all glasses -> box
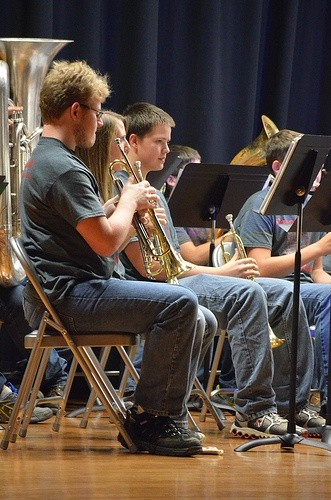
[79,104,103,119]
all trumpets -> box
[111,139,194,287]
[220,212,285,348]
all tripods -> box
[235,135,330,455]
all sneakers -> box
[117,414,202,455]
[231,409,308,441]
[279,409,325,436]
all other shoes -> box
[111,400,135,415]
[10,385,44,401]
[0,391,53,423]
[35,398,67,415]
[185,396,208,412]
[189,429,205,441]
[210,391,237,414]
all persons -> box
[230,129,331,426]
[0,107,70,424]
[160,144,326,438]
[18,60,220,456]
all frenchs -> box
[207,113,278,239]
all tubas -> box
[0,37,75,288]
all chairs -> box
[0,236,228,455]
